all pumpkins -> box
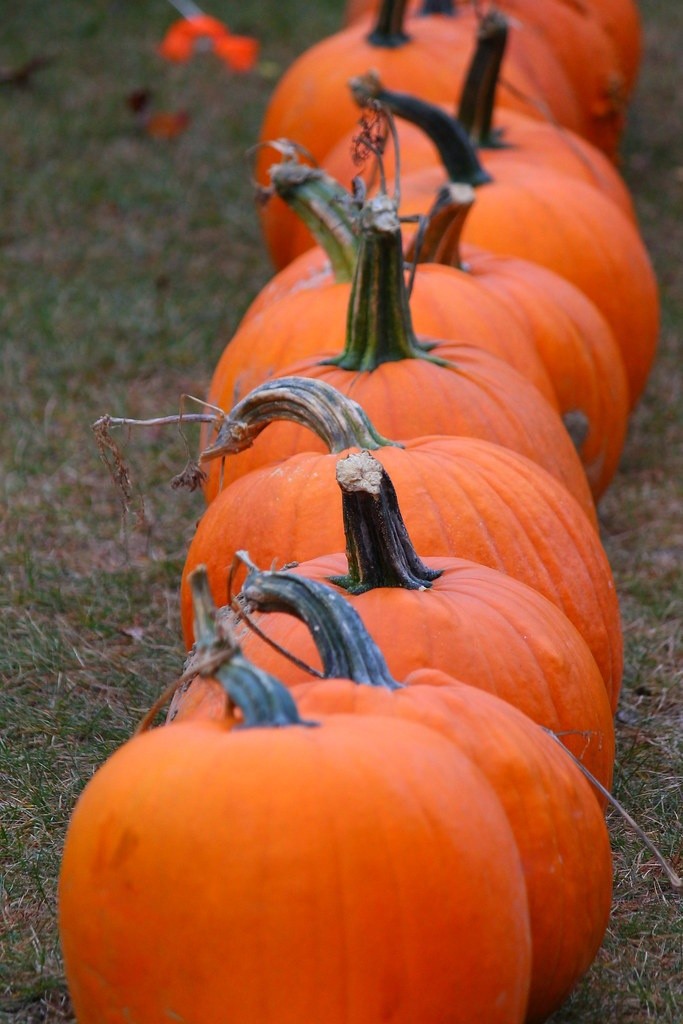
[59,0,660,1024]
